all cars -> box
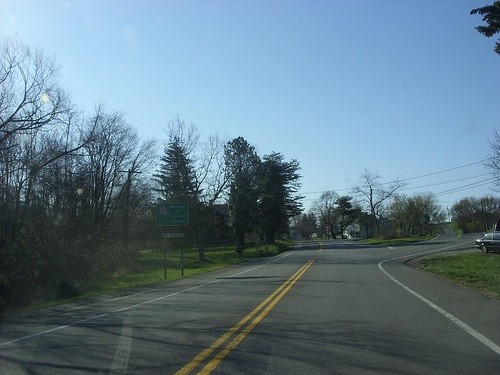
[476,231,500,255]
